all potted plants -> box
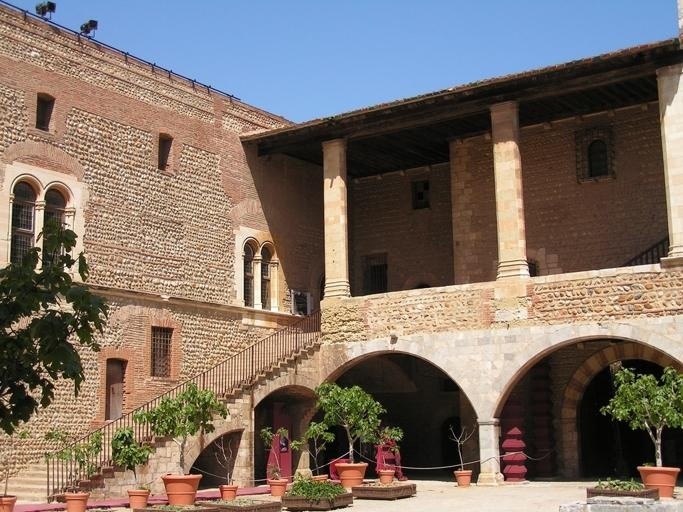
[584,476,661,501]
[260,426,288,497]
[214,435,238,501]
[110,426,154,510]
[281,383,417,509]
[448,425,473,488]
[43,428,104,511]
[596,363,683,498]
[132,384,230,506]
[1,416,34,511]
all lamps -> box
[77,19,98,40]
[34,0,56,23]
